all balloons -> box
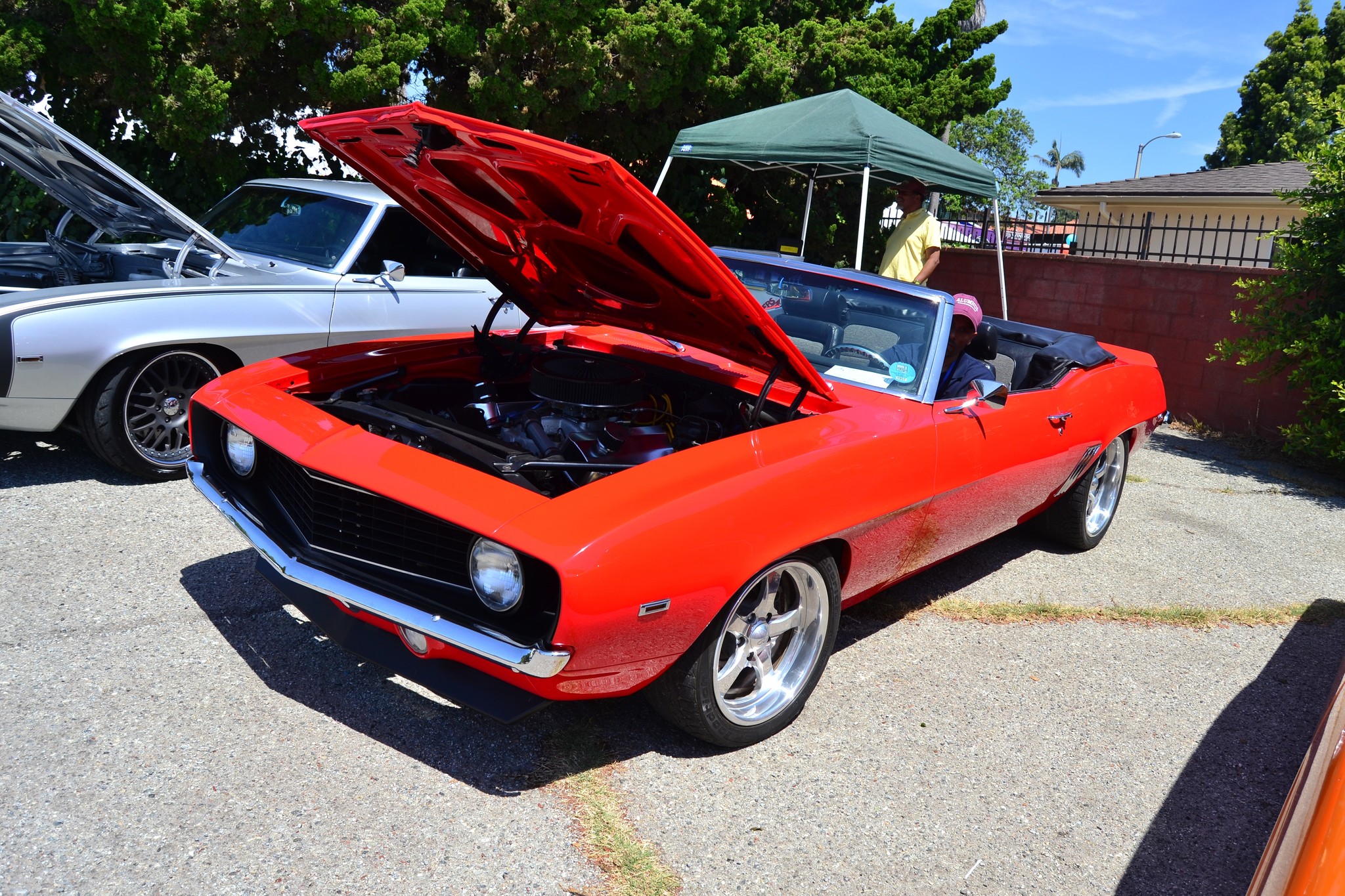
[1066,234,1076,245]
[1059,244,1070,254]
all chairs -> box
[772,284,851,361]
[921,312,1000,382]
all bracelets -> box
[912,279,921,285]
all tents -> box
[652,89,1009,321]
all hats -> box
[952,293,983,332]
[889,179,928,194]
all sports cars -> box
[0,88,583,485]
[181,99,1169,751]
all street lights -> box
[1134,131,1182,178]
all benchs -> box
[839,305,1057,387]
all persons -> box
[870,293,995,399]
[877,179,941,286]
